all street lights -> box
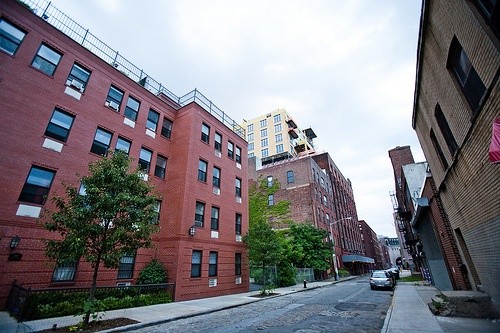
[329,217,352,281]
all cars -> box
[387,267,400,286]
[369,271,393,291]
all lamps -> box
[190,224,196,237]
[8,235,23,260]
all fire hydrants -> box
[303,280,307,288]
